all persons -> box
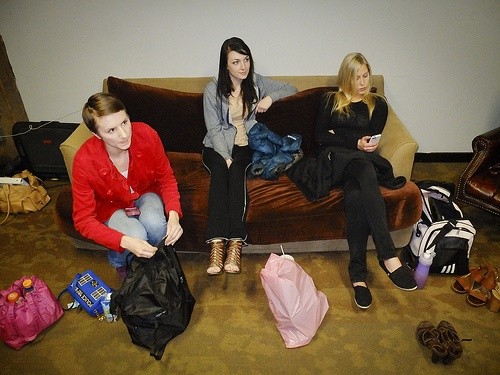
[316,52,417,309]
[203,37,300,274]
[72,92,184,273]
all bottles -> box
[22,279,34,296]
[414,252,434,289]
[7,290,24,305]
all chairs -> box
[455,125,500,215]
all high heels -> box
[438,321,473,358]
[416,321,453,364]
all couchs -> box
[58,75,423,255]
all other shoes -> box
[353,285,372,308]
[379,259,417,291]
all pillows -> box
[107,76,207,153]
[263,86,377,151]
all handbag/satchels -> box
[259,254,329,349]
[108,236,195,361]
[0,170,51,225]
[0,274,63,350]
[404,181,476,275]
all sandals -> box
[450,265,489,294]
[224,240,241,274]
[207,239,225,275]
[466,266,500,307]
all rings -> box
[147,256,148,258]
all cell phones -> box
[369,134,381,145]
